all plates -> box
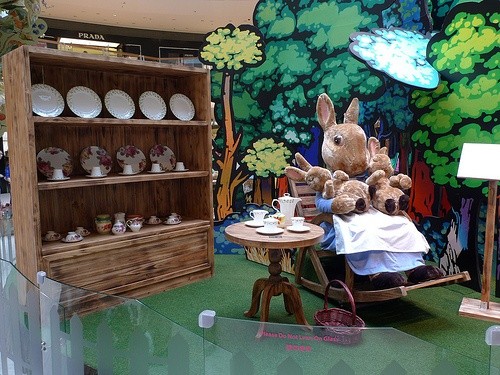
[163,221,181,224]
[169,94,195,120]
[37,147,73,177]
[104,90,135,119]
[80,146,113,175]
[116,145,146,173]
[86,175,107,178]
[147,171,165,173]
[42,236,62,241]
[139,91,167,120]
[145,220,161,224]
[118,172,137,175]
[79,232,91,237]
[31,84,65,117]
[245,220,264,227]
[173,169,189,171]
[47,177,70,180]
[287,226,310,232]
[150,144,176,170]
[257,227,284,234]
[66,86,102,118]
[62,237,83,242]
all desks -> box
[225,222,325,340]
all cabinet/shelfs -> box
[1,44,215,323]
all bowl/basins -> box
[149,216,159,223]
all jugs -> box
[126,218,144,232]
[272,193,302,224]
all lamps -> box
[456,143,500,323]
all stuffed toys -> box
[316,93,444,291]
[285,152,376,214]
[365,137,412,215]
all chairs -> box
[287,178,471,302]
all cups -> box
[66,232,81,240]
[171,213,182,219]
[151,164,162,171]
[91,167,103,176]
[76,227,88,234]
[52,169,64,178]
[123,165,132,173]
[263,218,278,230]
[167,216,179,223]
[45,231,60,239]
[291,217,305,228]
[176,162,184,170]
[250,210,269,221]
